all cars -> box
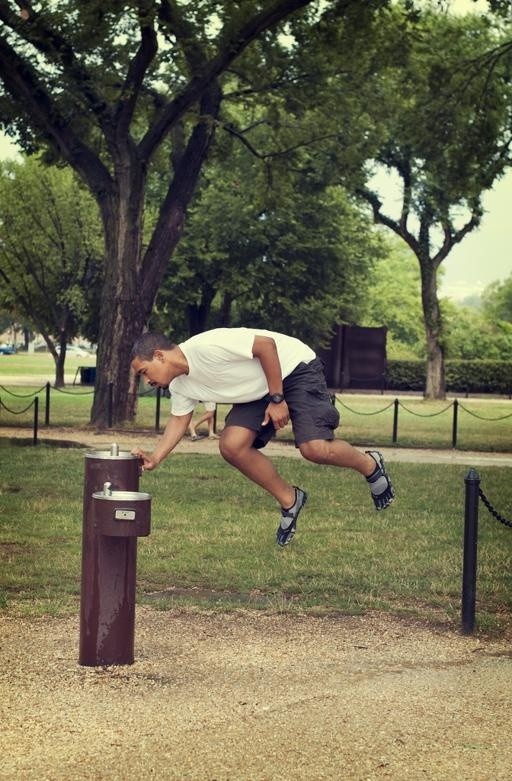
[55,344,89,359]
[0,344,16,353]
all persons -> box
[187,401,221,440]
[130,326,395,547]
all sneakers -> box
[363,450,396,512]
[188,427,221,442]
[274,486,307,549]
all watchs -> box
[270,393,285,404]
